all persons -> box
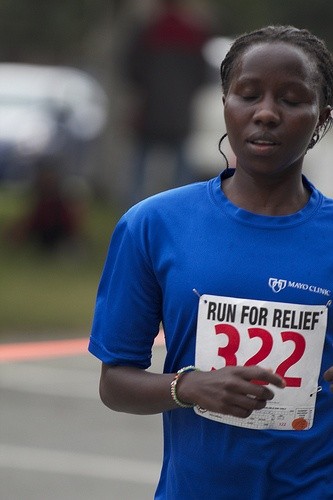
[99,25,333,500]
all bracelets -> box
[170,365,201,409]
